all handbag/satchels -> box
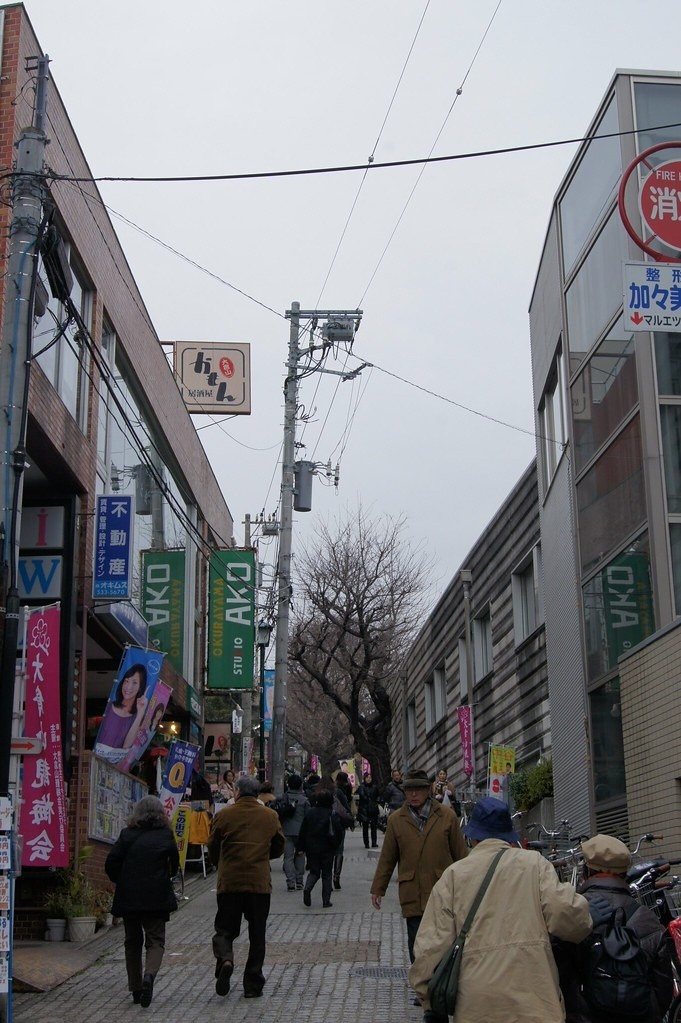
[442,792,461,818]
[333,791,348,821]
[427,933,471,1016]
[274,794,298,818]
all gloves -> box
[587,896,614,929]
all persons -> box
[410,797,593,1023]
[217,770,235,798]
[136,703,164,759]
[105,796,181,1008]
[341,761,350,774]
[98,664,150,750]
[433,769,456,810]
[355,774,381,848]
[259,771,355,907]
[209,777,286,997]
[550,833,674,1023]
[370,770,470,963]
[384,771,406,813]
[501,763,514,811]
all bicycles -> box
[510,809,681,1023]
[459,799,476,848]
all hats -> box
[582,833,634,875]
[462,797,519,845]
[398,769,430,787]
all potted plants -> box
[97,889,117,925]
[66,872,97,942]
[43,889,69,942]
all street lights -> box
[256,617,274,784]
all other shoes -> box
[333,878,341,890]
[140,974,155,1008]
[133,991,140,1004]
[323,901,332,907]
[215,961,232,995]
[288,888,295,892]
[245,990,264,997]
[296,885,302,889]
[373,843,378,847]
[304,890,311,906]
[365,843,369,848]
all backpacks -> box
[579,894,661,1023]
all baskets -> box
[541,829,570,846]
[626,882,681,920]
